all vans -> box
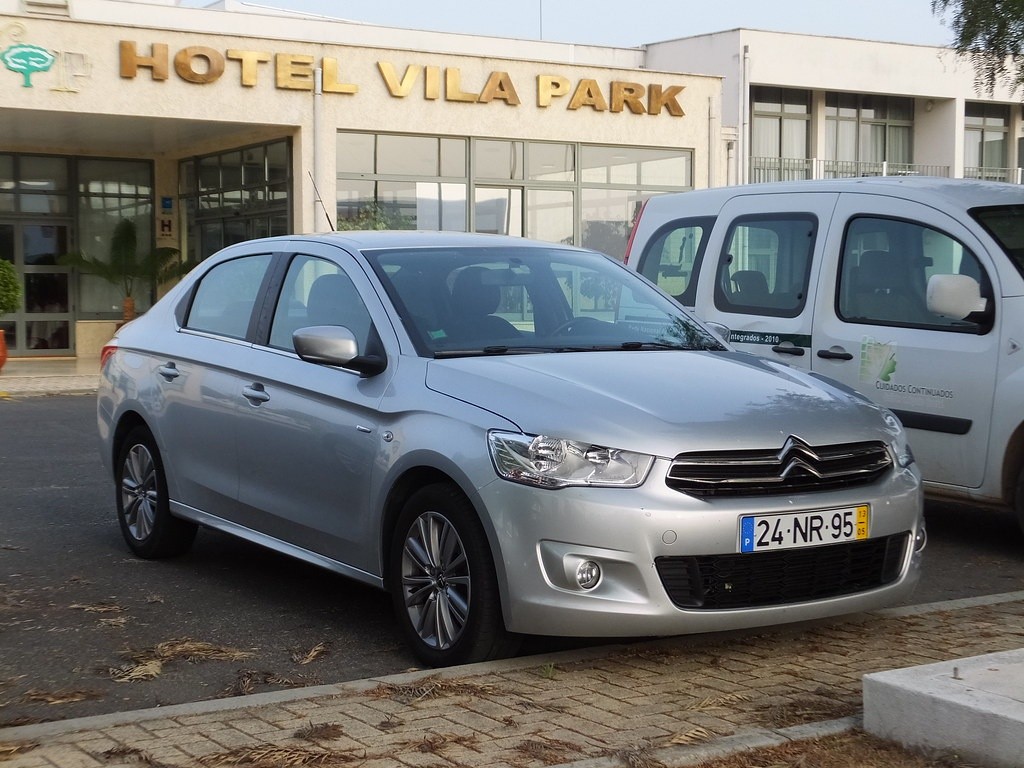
[622,174,1024,546]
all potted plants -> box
[0,259,22,369]
[55,219,199,356]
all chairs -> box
[392,269,453,340]
[852,250,930,320]
[727,270,772,306]
[453,265,524,339]
[308,274,382,353]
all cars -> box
[96,229,928,667]
